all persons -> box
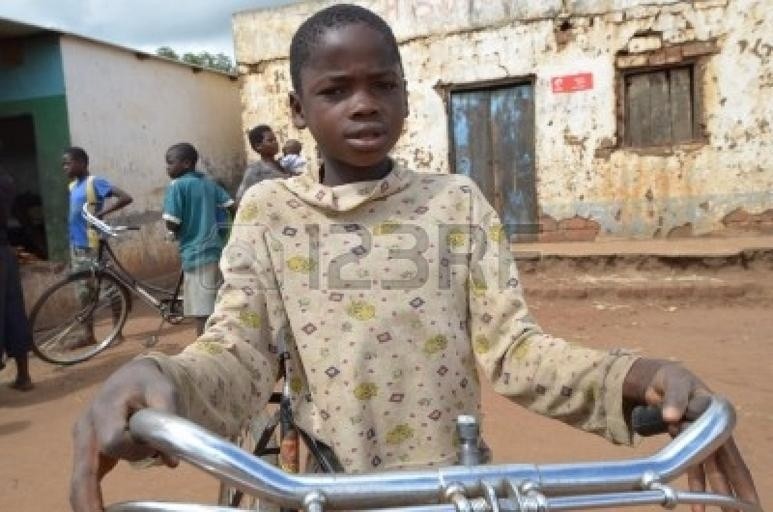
[158,142,237,345]
[0,161,36,394]
[233,125,291,210]
[62,143,135,351]
[279,137,308,177]
[62,2,759,510]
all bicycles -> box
[94,390,750,512]
[27,201,191,367]
